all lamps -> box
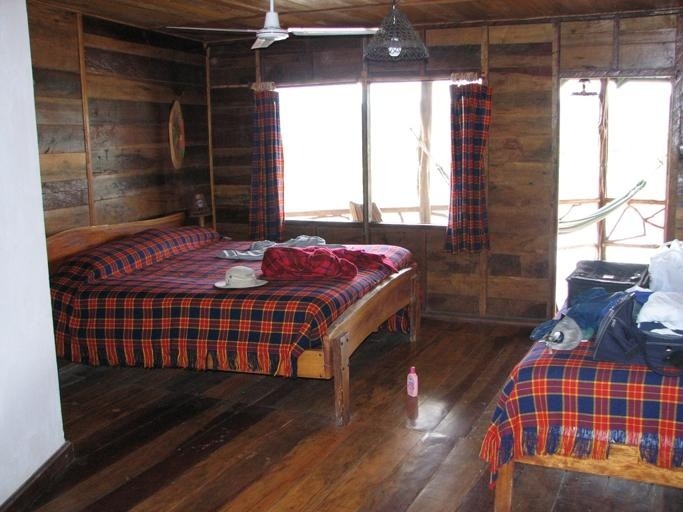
[187,195,211,226]
[363,1,430,63]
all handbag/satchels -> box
[592,290,683,377]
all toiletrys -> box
[407,365,419,398]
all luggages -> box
[565,260,650,310]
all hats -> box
[214,266,269,289]
[545,312,582,351]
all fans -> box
[166,0,386,50]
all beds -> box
[479,297,683,512]
[45,211,423,425]
